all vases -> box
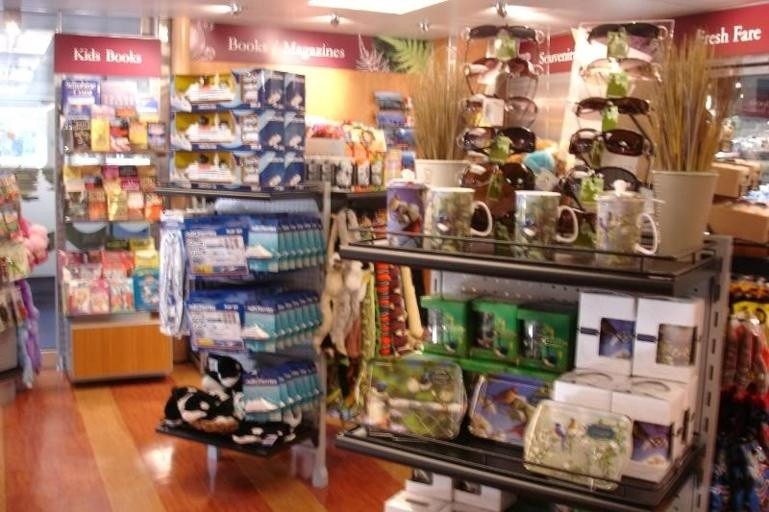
[411,156,472,194]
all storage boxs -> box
[168,59,311,194]
[354,287,708,491]
[383,466,519,511]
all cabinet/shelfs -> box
[332,223,740,512]
[43,30,176,386]
[309,182,387,492]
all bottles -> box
[413,157,472,236]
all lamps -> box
[331,11,344,27]
[494,0,508,20]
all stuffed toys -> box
[315,206,424,426]
[160,352,303,450]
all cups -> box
[384,180,425,235]
[513,186,582,249]
[429,186,493,241]
[593,177,660,258]
